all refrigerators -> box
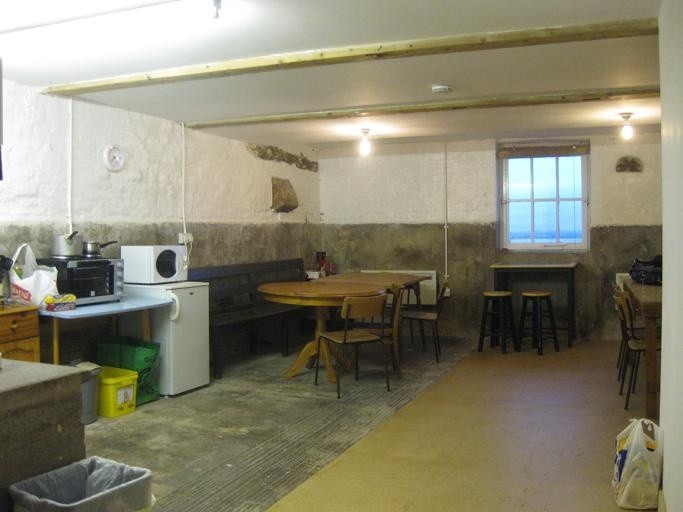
[119,281,209,396]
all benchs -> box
[186,259,312,380]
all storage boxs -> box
[98,365,138,419]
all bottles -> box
[305,249,337,278]
[12,259,22,279]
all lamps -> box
[620,113,633,141]
[358,129,372,157]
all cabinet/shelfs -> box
[0,303,41,363]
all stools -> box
[517,291,560,356]
[477,290,516,355]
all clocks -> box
[104,145,125,171]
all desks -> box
[489,262,578,349]
[38,295,173,365]
[257,280,387,360]
[311,271,432,350]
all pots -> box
[47,231,118,257]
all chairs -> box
[610,280,661,410]
[314,293,390,398]
[352,287,404,381]
[400,286,446,363]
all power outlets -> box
[178,233,193,244]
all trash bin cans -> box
[8,456,152,512]
[67,359,102,424]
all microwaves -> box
[36,257,124,306]
[120,245,189,285]
